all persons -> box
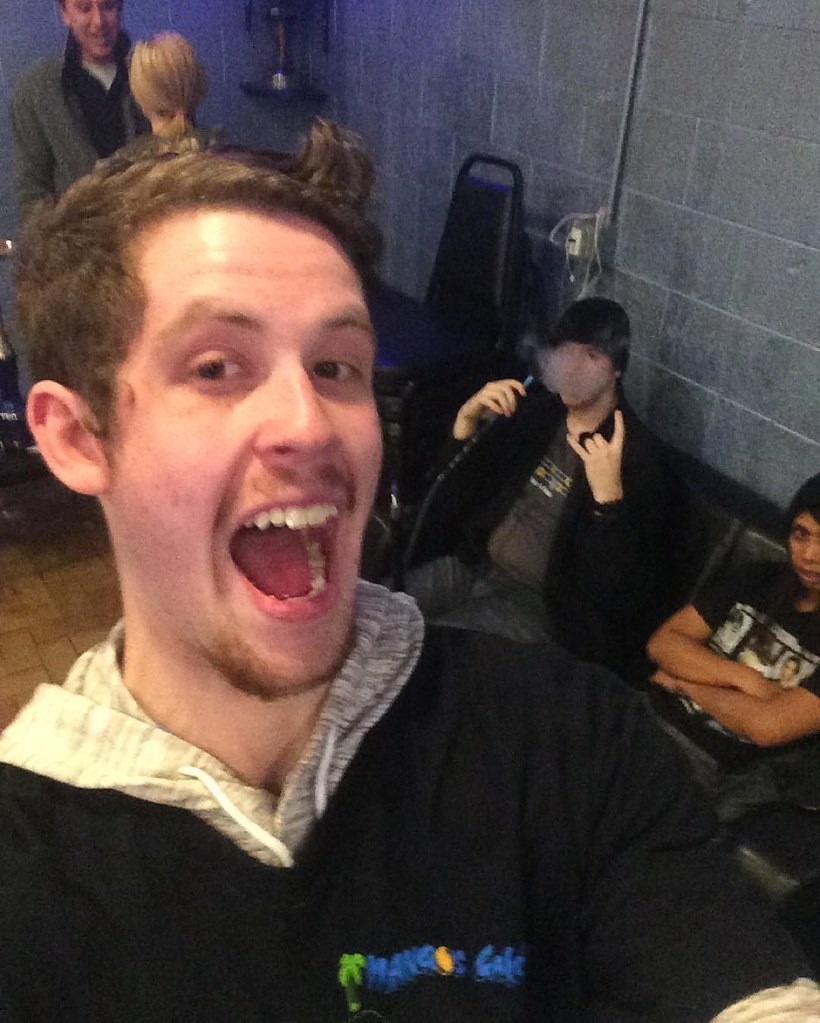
[389,296,696,680]
[8,0,150,226]
[123,30,212,151]
[639,474,820,813]
[0,115,820,1023]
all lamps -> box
[239,1,333,105]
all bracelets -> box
[592,499,623,514]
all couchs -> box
[655,450,820,916]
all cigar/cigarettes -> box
[518,373,534,389]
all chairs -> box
[371,150,523,427]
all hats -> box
[551,298,630,369]
